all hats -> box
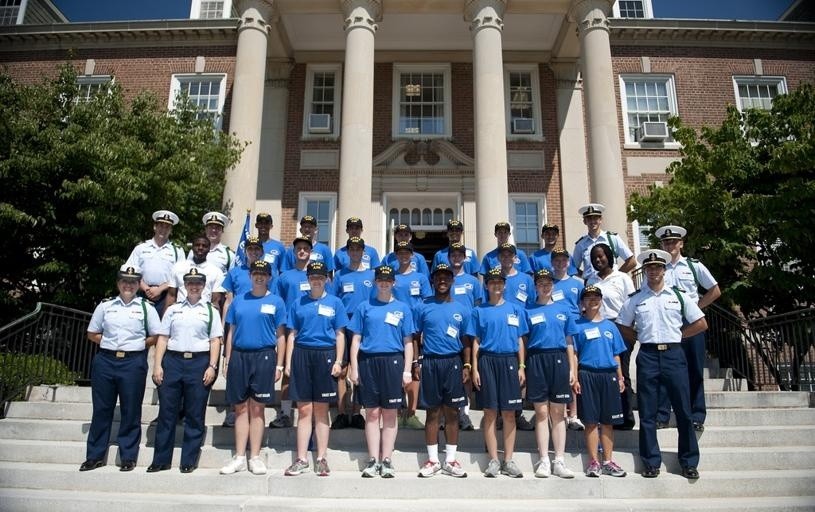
[244,237,264,253]
[581,285,603,300]
[551,247,569,259]
[430,263,455,281]
[346,236,365,251]
[255,211,273,228]
[201,210,229,227]
[152,210,180,226]
[394,224,411,235]
[448,242,466,257]
[115,264,143,282]
[293,235,313,248]
[447,220,464,233]
[183,264,207,282]
[499,242,516,256]
[249,259,271,274]
[346,216,363,233]
[306,261,328,276]
[534,269,554,283]
[394,240,413,254]
[494,222,510,235]
[299,214,317,226]
[484,268,507,284]
[541,222,559,235]
[577,203,606,219]
[375,266,395,282]
[654,224,688,246]
[636,248,672,266]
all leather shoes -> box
[643,463,660,478]
[179,463,198,473]
[655,421,670,430]
[146,463,171,473]
[79,458,105,471]
[695,420,703,433]
[682,464,699,479]
[119,462,135,471]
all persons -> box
[127,210,186,321]
[333,218,381,272]
[619,250,708,480]
[270,237,331,428]
[551,247,587,431]
[471,268,530,478]
[410,262,476,478]
[430,242,483,430]
[585,243,639,432]
[285,262,351,476]
[382,241,432,431]
[573,286,628,477]
[147,265,224,472]
[80,264,160,471]
[222,240,280,428]
[149,234,224,427]
[529,224,578,279]
[478,223,535,284]
[381,223,431,281]
[654,225,722,432]
[348,265,416,477]
[574,203,637,286]
[236,213,290,275]
[523,268,579,479]
[483,243,536,430]
[326,238,378,430]
[431,218,481,281]
[186,211,235,321]
[286,215,336,283]
[218,261,288,474]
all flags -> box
[235,209,252,271]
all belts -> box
[94,344,149,358]
[640,342,680,352]
[164,349,211,358]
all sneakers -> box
[417,461,442,477]
[218,456,249,475]
[380,458,396,478]
[360,457,380,478]
[442,460,468,478]
[501,461,523,479]
[225,411,236,426]
[459,411,473,429]
[535,458,553,478]
[349,413,366,429]
[484,460,502,478]
[585,463,602,480]
[269,414,294,428]
[552,462,574,480]
[248,456,267,474]
[330,413,349,430]
[314,456,330,476]
[405,412,425,430]
[602,461,627,477]
[284,459,310,477]
[518,415,535,431]
[569,417,585,431]
